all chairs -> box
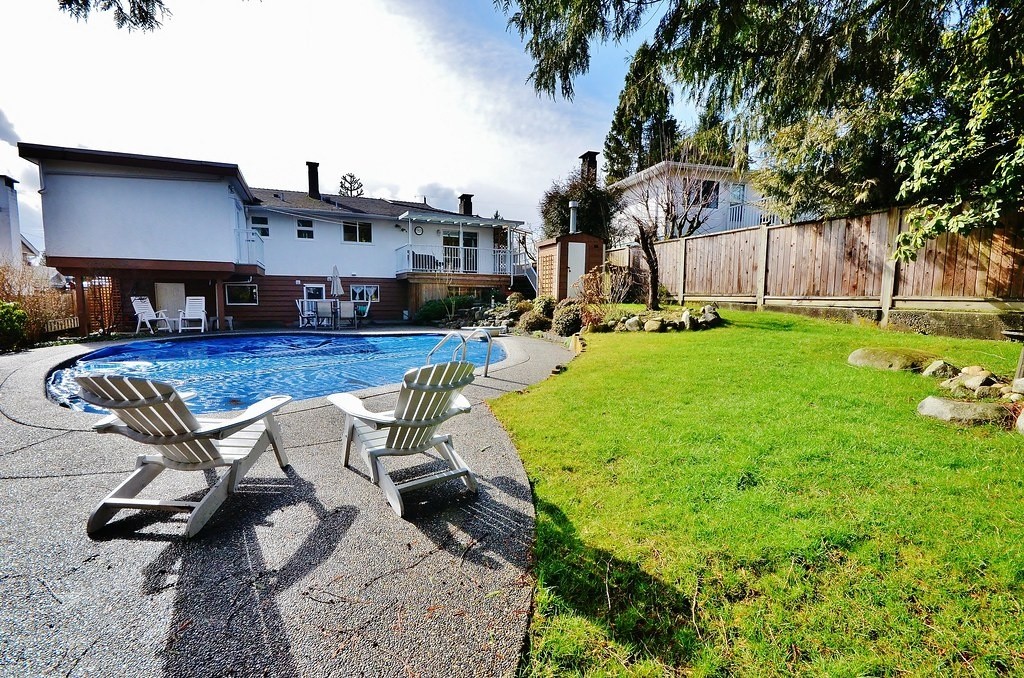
[74,374,293,539]
[131,296,172,335]
[327,360,478,517]
[295,298,357,331]
[178,296,208,333]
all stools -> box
[211,316,233,331]
